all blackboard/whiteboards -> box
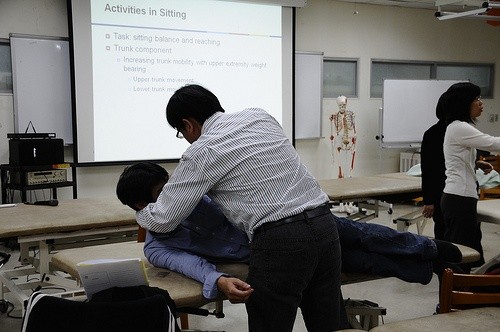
[8,31,324,148]
[382,78,471,143]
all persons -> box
[115,162,463,306]
[136,85,349,332]
[441,82,500,270]
[422,92,493,240]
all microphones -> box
[24,198,59,206]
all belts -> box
[254,205,330,235]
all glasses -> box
[475,96,481,102]
[176,121,183,138]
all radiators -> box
[399,151,421,173]
